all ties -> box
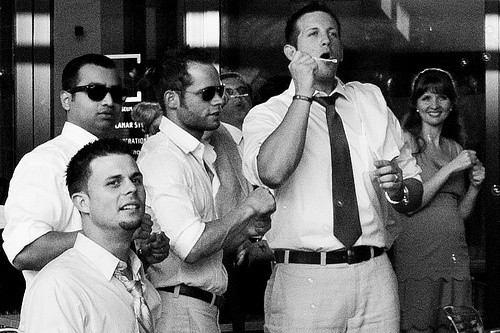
[310,94,363,249]
[123,275,156,333]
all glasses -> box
[69,83,127,104]
[174,84,224,101]
[224,85,251,96]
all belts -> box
[156,282,225,309]
[272,245,386,265]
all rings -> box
[394,173,400,182]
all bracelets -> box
[385,186,410,208]
[291,94,313,105]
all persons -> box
[392,67,486,333]
[136,44,276,333]
[240,3,424,333]
[129,73,255,138]
[3,52,170,289]
[18,137,163,333]
